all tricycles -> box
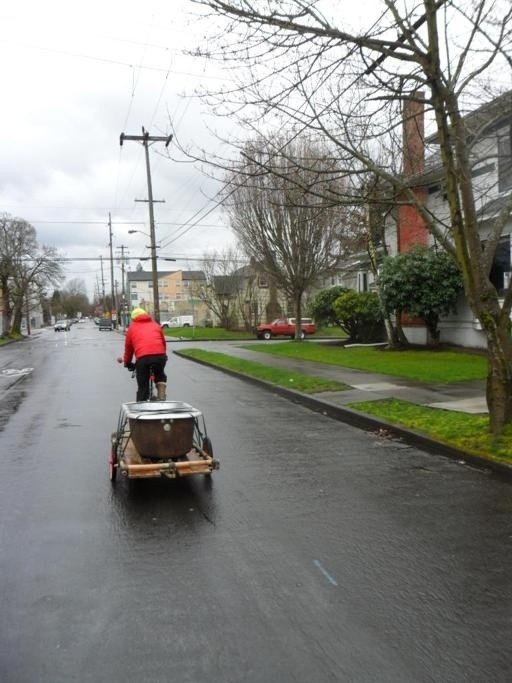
[108,355,221,495]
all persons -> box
[123,306,168,400]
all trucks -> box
[161,313,194,329]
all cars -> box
[93,316,113,330]
[55,316,87,331]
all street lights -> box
[128,228,160,324]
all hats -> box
[130,307,148,319]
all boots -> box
[155,382,168,401]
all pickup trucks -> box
[254,318,317,339]
